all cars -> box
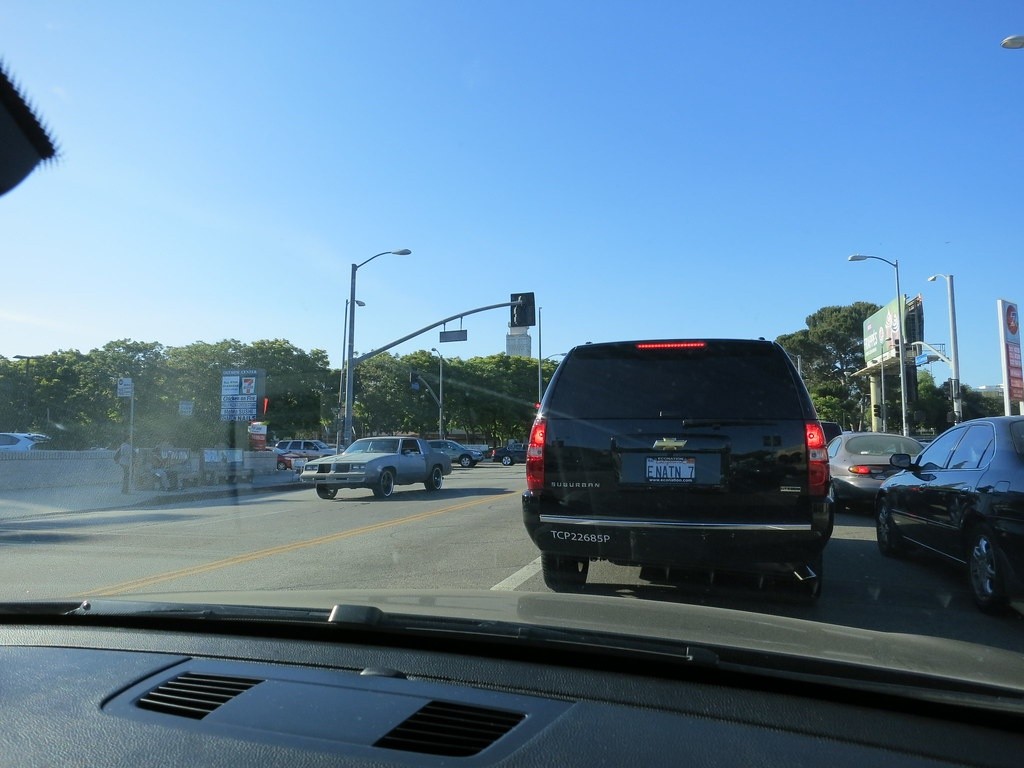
[826,432,927,511]
[266,447,299,471]
[873,415,1024,613]
[491,443,529,466]
[300,437,453,502]
[426,440,484,468]
[0,432,52,451]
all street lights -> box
[927,274,963,428]
[344,247,411,448]
[848,254,909,436]
[13,355,44,387]
[430,347,443,440]
[335,298,365,459]
[880,336,891,433]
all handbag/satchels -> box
[114,448,121,464]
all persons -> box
[118,435,139,494]
[151,441,184,490]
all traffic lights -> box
[894,339,900,358]
[874,404,884,418]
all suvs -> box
[273,440,338,462]
[522,337,835,605]
[821,422,843,446]
